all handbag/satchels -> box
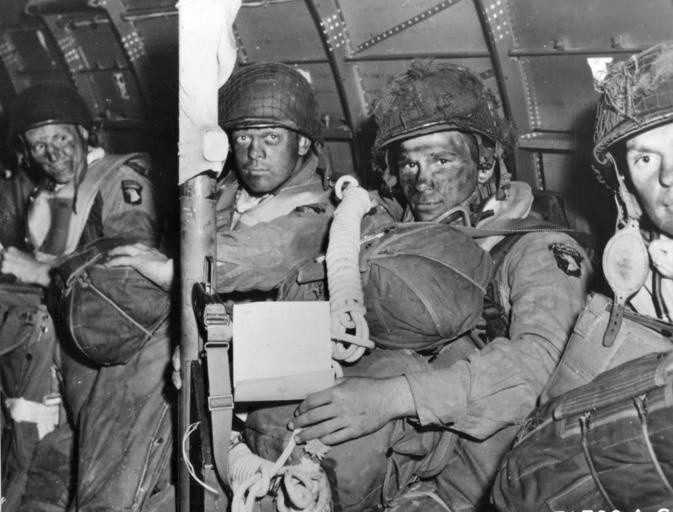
[489,291,672,512]
[273,222,498,353]
[242,324,494,510]
[42,236,173,367]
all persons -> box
[487,33,671,512]
[283,57,596,512]
[0,80,164,512]
[21,59,354,512]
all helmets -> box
[368,59,515,169]
[590,38,672,193]
[218,60,326,149]
[8,81,94,138]
